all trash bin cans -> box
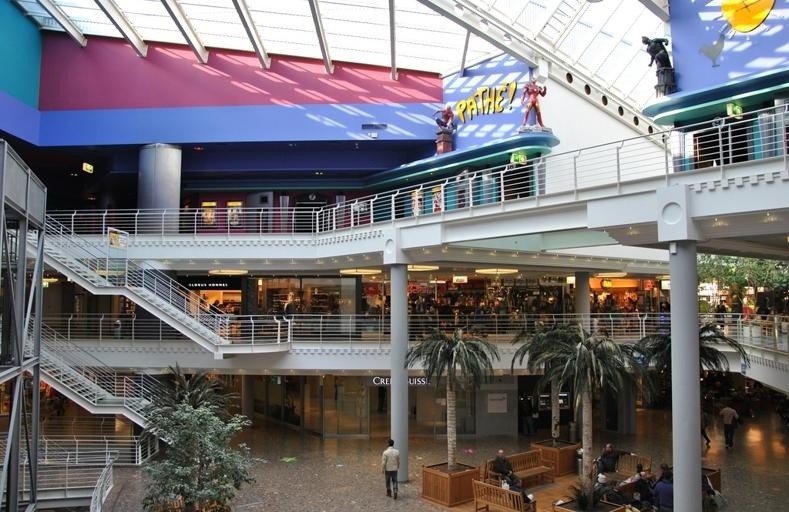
[576,448,583,475]
[568,422,578,443]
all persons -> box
[702,472,721,508]
[630,492,656,511]
[755,295,772,320]
[599,442,637,473]
[716,299,727,328]
[651,470,673,511]
[642,35,674,73]
[432,104,457,135]
[615,463,648,488]
[517,76,548,131]
[176,203,194,235]
[508,477,534,511]
[651,462,672,490]
[701,405,711,446]
[636,470,656,507]
[209,299,220,315]
[381,438,400,500]
[719,401,740,449]
[494,448,523,490]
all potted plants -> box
[627,321,752,511]
[696,254,789,339]
[402,319,501,510]
[507,320,609,511]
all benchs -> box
[485,448,556,488]
[472,478,537,511]
[611,474,656,501]
[604,453,652,480]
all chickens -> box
[700,34,726,67]
